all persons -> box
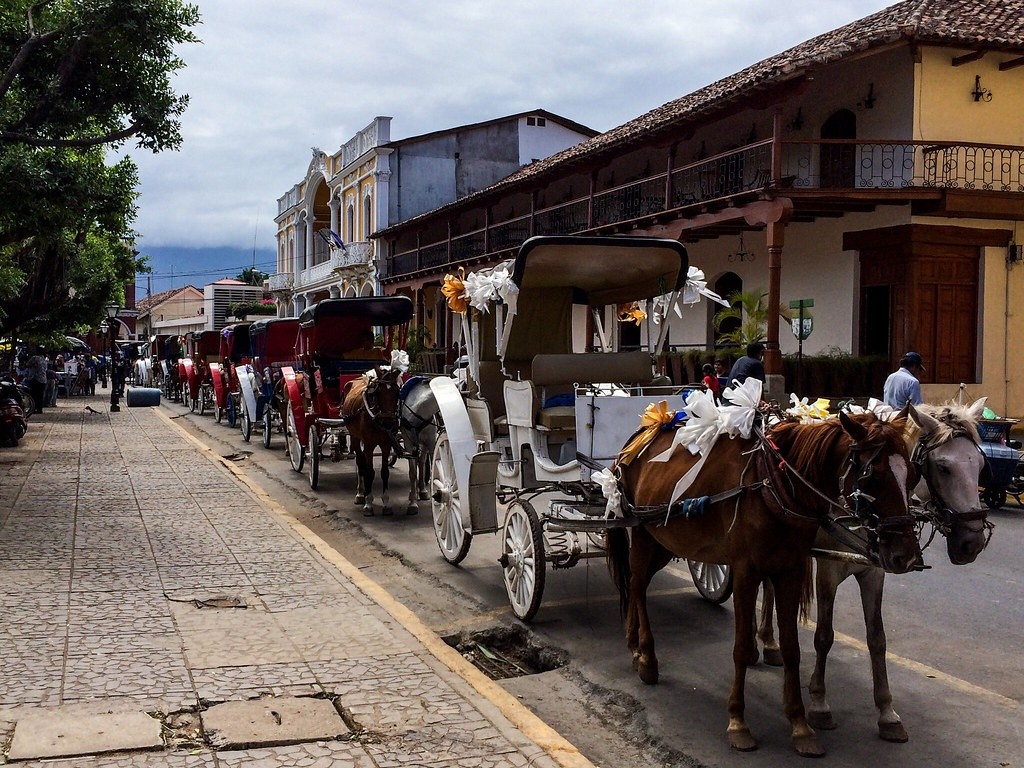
[721,341,765,408]
[26,347,100,414]
[883,351,925,412]
[702,359,729,399]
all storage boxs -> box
[977,442,1019,490]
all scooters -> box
[0,373,28,448]
[17,383,35,420]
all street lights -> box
[99,319,109,388]
[104,304,121,412]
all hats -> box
[906,352,927,372]
[138,354,143,356]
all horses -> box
[277,373,349,456]
[604,401,924,758]
[343,364,460,517]
[747,396,989,746]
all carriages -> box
[118,235,997,756]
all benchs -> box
[164,352,302,390]
[471,362,510,436]
[320,358,389,406]
[531,353,656,427]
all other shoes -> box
[88,393,94,396]
[45,404,50,407]
[33,410,43,414]
[52,404,57,407]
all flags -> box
[316,228,346,254]
